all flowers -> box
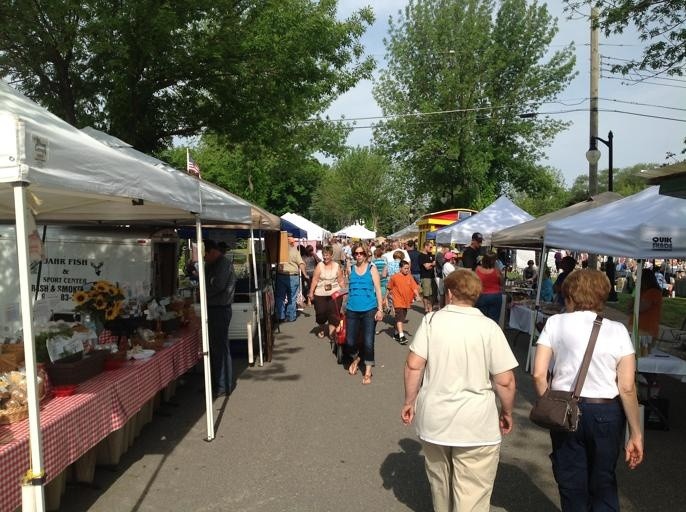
[73,280,127,320]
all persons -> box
[270,233,512,384]
[523,251,685,354]
[201,239,237,397]
[532,267,645,512]
[400,269,520,511]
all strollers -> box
[330,288,348,365]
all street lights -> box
[585,130,614,192]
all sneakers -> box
[399,336,408,343]
[393,333,399,340]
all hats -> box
[451,248,460,254]
[444,252,455,259]
[472,232,484,241]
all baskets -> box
[0,393,46,425]
[0,344,25,375]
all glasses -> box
[354,252,364,255]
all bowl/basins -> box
[49,386,76,397]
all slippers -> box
[362,375,371,385]
[318,331,325,338]
[349,362,359,375]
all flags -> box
[187,153,202,179]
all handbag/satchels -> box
[530,388,581,433]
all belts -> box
[579,397,615,404]
[279,273,298,276]
